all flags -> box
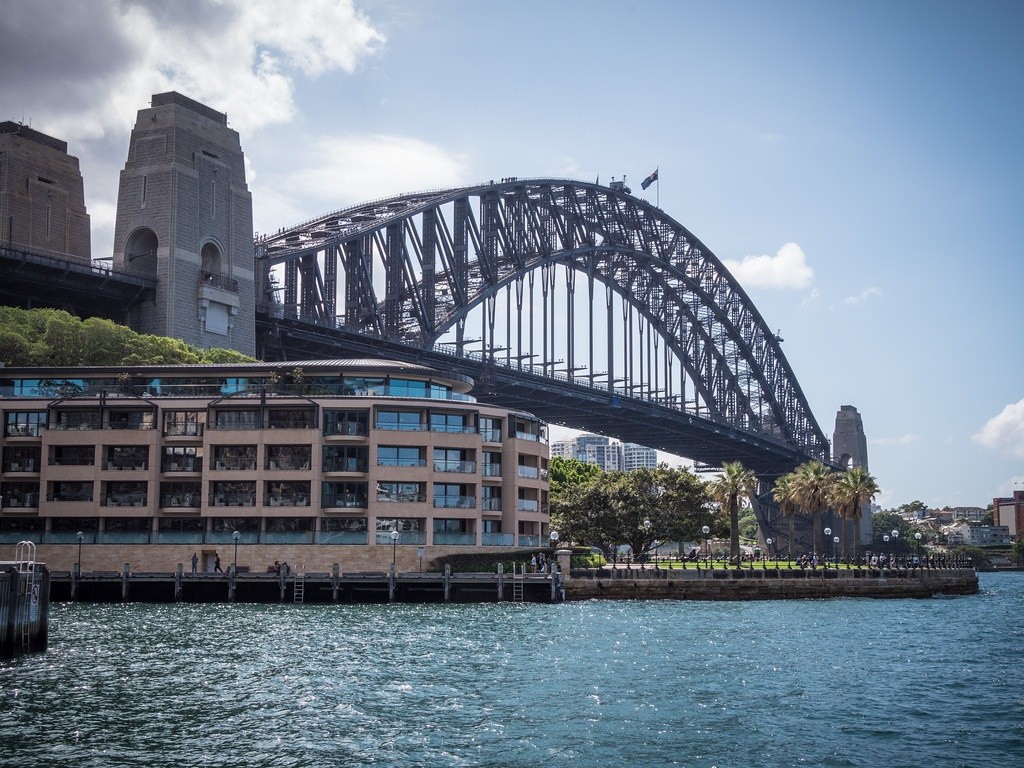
[641,169,658,190]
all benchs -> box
[226,566,249,571]
[267,566,289,573]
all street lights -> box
[883,535,889,558]
[391,531,399,565]
[702,526,710,568]
[232,531,240,578]
[824,528,832,568]
[766,538,772,553]
[550,531,559,564]
[644,520,650,562]
[892,530,899,568]
[76,531,84,577]
[915,533,922,568]
[833,537,839,568]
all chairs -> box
[4,419,369,512]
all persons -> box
[191,553,198,572]
[690,549,698,562]
[274,560,291,576]
[214,553,223,573]
[531,553,551,573]
[553,559,561,572]
[796,551,919,569]
